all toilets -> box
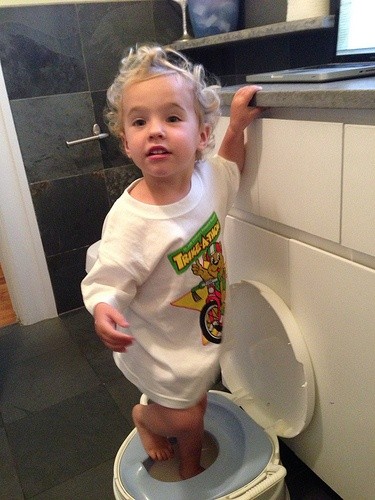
[111,276,315,499]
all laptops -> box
[245,0,375,83]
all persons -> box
[81,45,270,480]
